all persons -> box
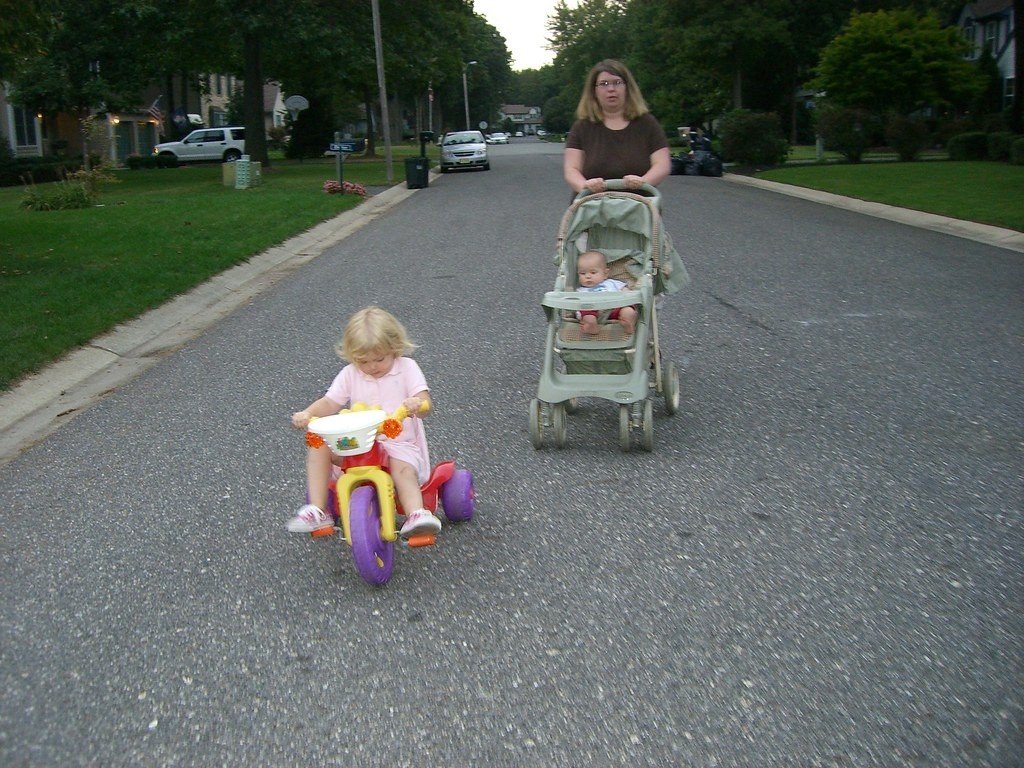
[575,250,639,334]
[563,59,672,369]
[287,307,442,537]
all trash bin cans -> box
[405,156,429,188]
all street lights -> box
[461,60,478,130]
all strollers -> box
[528,178,681,453]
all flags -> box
[146,96,165,137]
[169,105,187,130]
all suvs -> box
[150,126,245,167]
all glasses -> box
[595,80,627,87]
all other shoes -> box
[286,503,335,532]
[399,509,442,539]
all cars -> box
[436,130,490,171]
[488,132,509,146]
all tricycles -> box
[297,400,476,588]
